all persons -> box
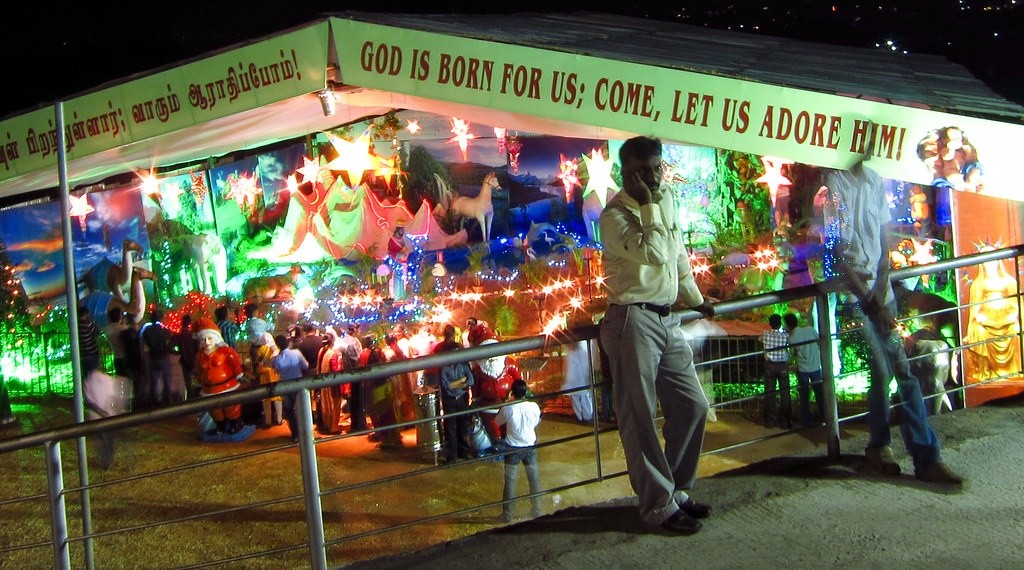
[825,112,965,486]
[564,340,593,421]
[963,244,1023,382]
[784,313,824,423]
[440,343,472,465]
[245,303,436,448]
[167,312,197,398]
[104,310,144,386]
[468,324,521,441]
[78,308,99,374]
[141,311,173,400]
[759,314,792,427]
[215,306,239,349]
[599,136,714,534]
[495,379,541,523]
[191,317,246,435]
[433,321,461,353]
[79,356,117,468]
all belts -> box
[631,302,672,317]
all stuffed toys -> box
[937,126,984,191]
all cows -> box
[242,262,306,301]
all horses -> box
[77,238,144,299]
[450,172,503,243]
[77,266,158,327]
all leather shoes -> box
[678,499,711,516]
[665,510,703,534]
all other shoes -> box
[280,419,413,449]
[498,514,510,522]
[865,445,901,477]
[782,421,794,429]
[766,422,774,427]
[914,460,964,485]
